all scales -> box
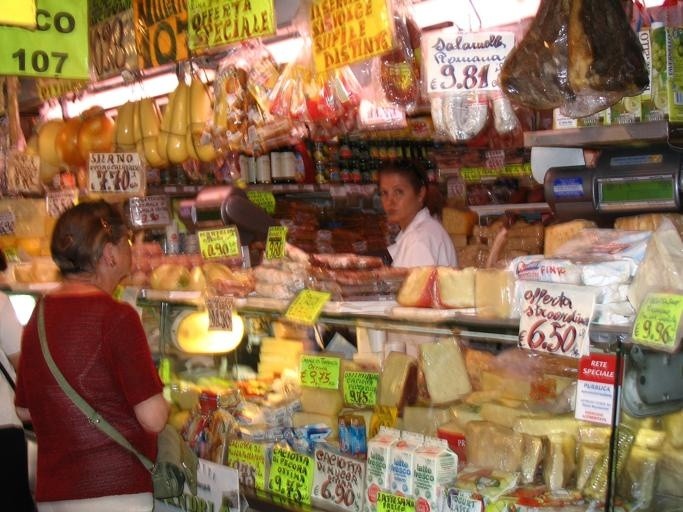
[523,119,683,222]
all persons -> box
[11,197,174,512]
[376,159,461,268]
[0,250,39,512]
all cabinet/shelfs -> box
[0,299,683,512]
[144,137,560,247]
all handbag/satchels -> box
[143,422,202,500]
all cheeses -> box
[173,214,683,512]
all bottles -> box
[235,137,439,185]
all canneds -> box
[312,135,448,185]
